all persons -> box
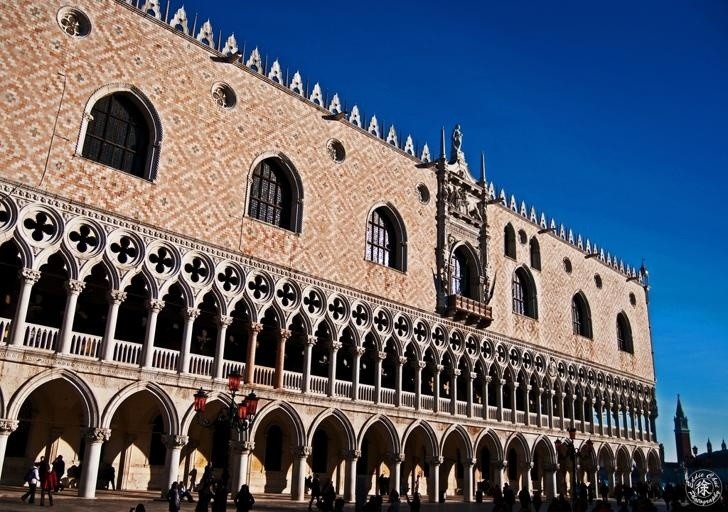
[129,504,145,512]
[106,464,115,491]
[21,455,65,506]
[452,124,463,152]
[306,473,421,512]
[491,479,728,512]
[166,461,255,512]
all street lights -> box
[193,364,260,511]
[555,421,595,512]
